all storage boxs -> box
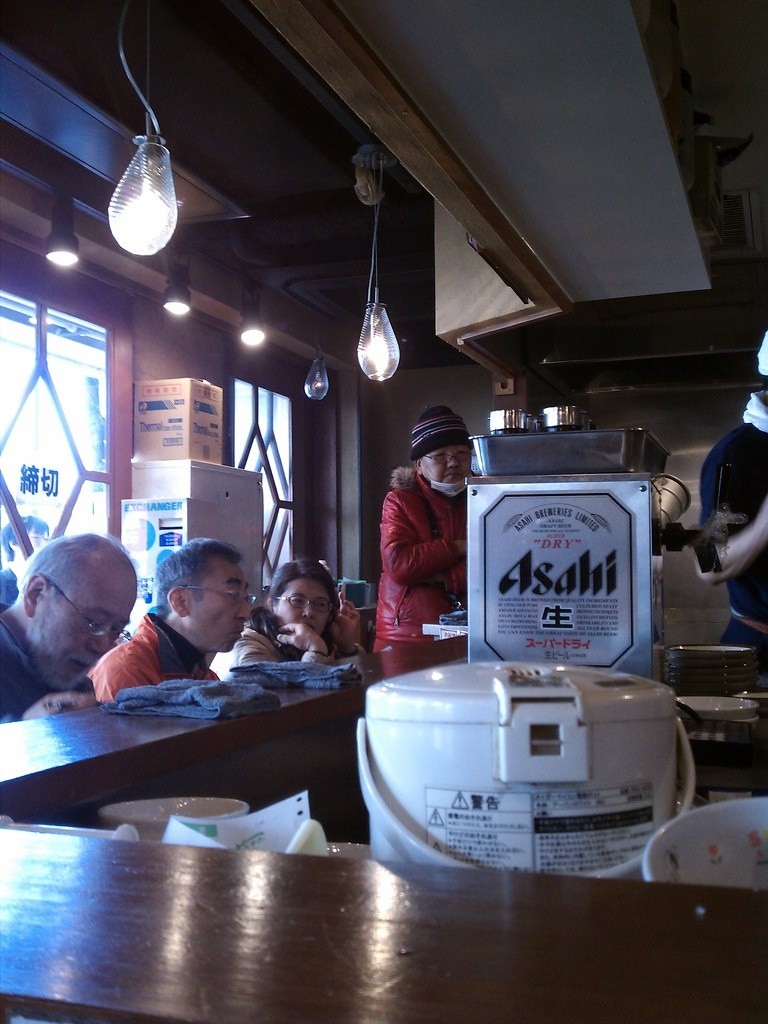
[131,377,225,466]
[119,497,221,631]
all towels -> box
[438,609,468,626]
[223,660,364,691]
[98,678,285,721]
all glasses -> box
[425,450,472,464]
[173,585,257,606]
[48,580,133,643]
[274,594,335,613]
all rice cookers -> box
[355,663,698,884]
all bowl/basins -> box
[641,794,767,891]
[490,405,597,434]
[661,641,768,728]
[97,795,250,841]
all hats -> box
[410,405,471,460]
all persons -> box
[87,536,252,705]
[0,535,136,723]
[373,406,475,651]
[0,517,48,606]
[210,559,366,679]
[698,332,768,674]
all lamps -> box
[163,237,192,316]
[108,0,178,256]
[303,332,329,401]
[355,137,401,383]
[239,277,266,347]
[44,190,80,267]
